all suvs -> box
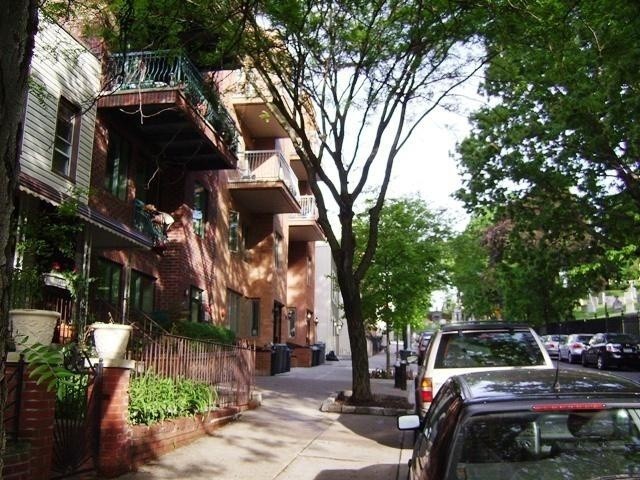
[411,319,556,424]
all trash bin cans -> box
[311,343,325,367]
[271,344,290,376]
[393,365,401,388]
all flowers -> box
[51,262,80,298]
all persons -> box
[128,180,144,206]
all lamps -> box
[306,312,312,321]
[313,317,319,326]
[284,311,292,320]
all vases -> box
[92,321,134,361]
[8,308,62,351]
[43,273,67,289]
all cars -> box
[417,331,435,356]
[540,333,570,360]
[579,331,640,371]
[396,367,640,480]
[556,333,595,363]
